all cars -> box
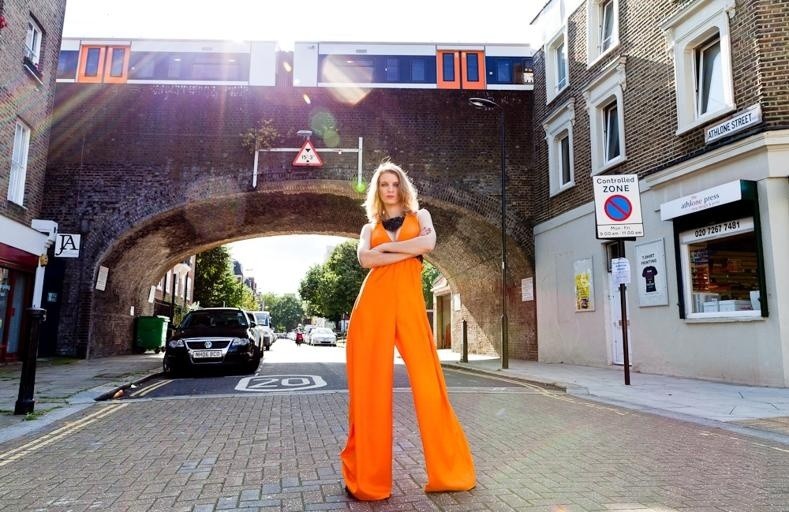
[279,326,337,346]
[164,307,275,376]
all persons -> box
[337,159,477,502]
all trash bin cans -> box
[136,315,170,349]
